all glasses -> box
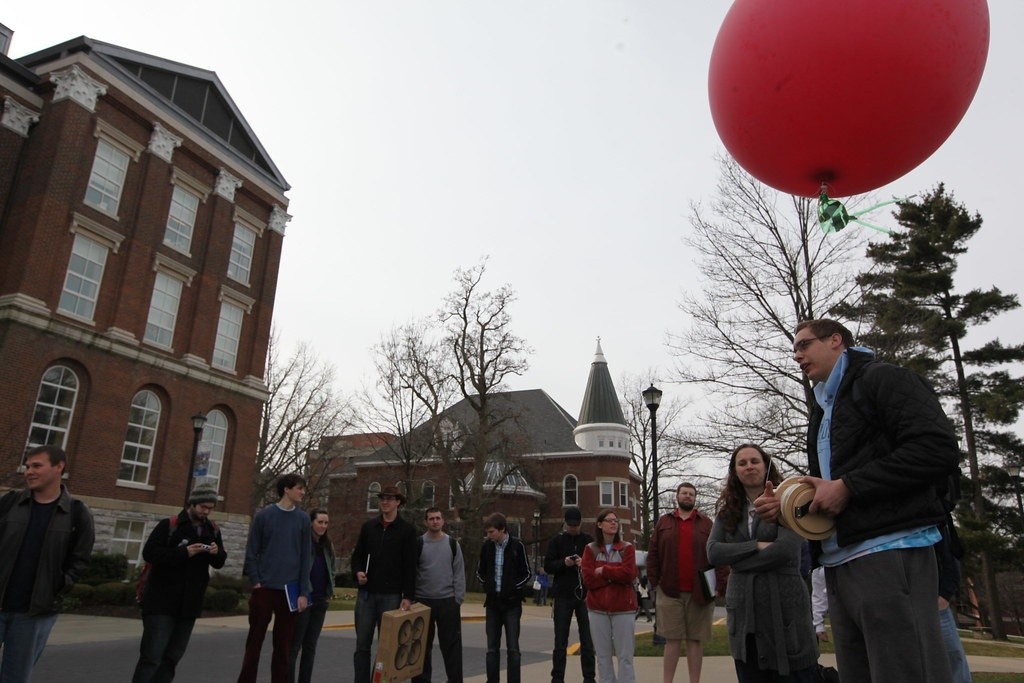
[679,492,694,496]
[792,334,832,353]
[604,518,619,522]
[199,504,213,510]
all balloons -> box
[710,0,989,198]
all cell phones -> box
[570,556,577,561]
[199,544,211,550]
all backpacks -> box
[136,516,219,604]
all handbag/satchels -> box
[533,580,541,591]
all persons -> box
[633,576,654,623]
[411,507,465,683]
[933,514,972,683]
[476,513,532,683]
[808,540,829,642]
[351,487,418,683]
[581,510,638,683]
[237,473,336,683]
[646,482,730,683]
[0,445,95,683]
[131,483,227,683]
[544,507,597,683]
[533,567,549,606]
[753,319,960,683]
[706,444,820,683]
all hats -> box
[187,482,218,507]
[376,487,407,505]
[565,507,581,526]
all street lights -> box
[534,507,541,573]
[184,411,208,509]
[642,382,666,645]
[1005,460,1024,530]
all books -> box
[284,582,313,612]
[699,567,717,602]
[363,554,370,581]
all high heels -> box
[647,619,652,622]
